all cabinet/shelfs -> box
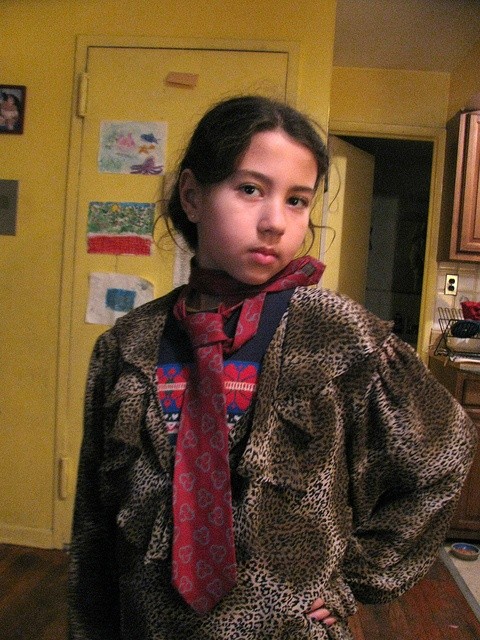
[437,107,480,263]
[429,355,480,540]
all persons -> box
[0,95,19,130]
[68,96,478,640]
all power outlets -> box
[445,274,458,296]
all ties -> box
[169,255,328,617]
